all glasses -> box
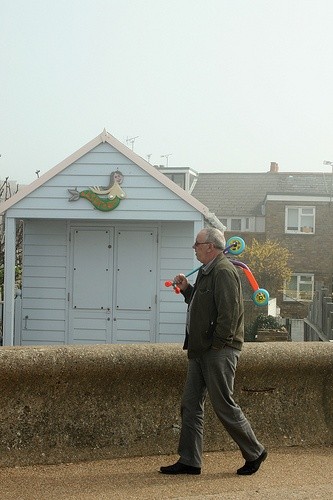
[193,241,212,247]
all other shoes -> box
[237,450,268,475]
[159,461,202,475]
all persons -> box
[160,228,267,475]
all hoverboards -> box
[165,236,270,307]
